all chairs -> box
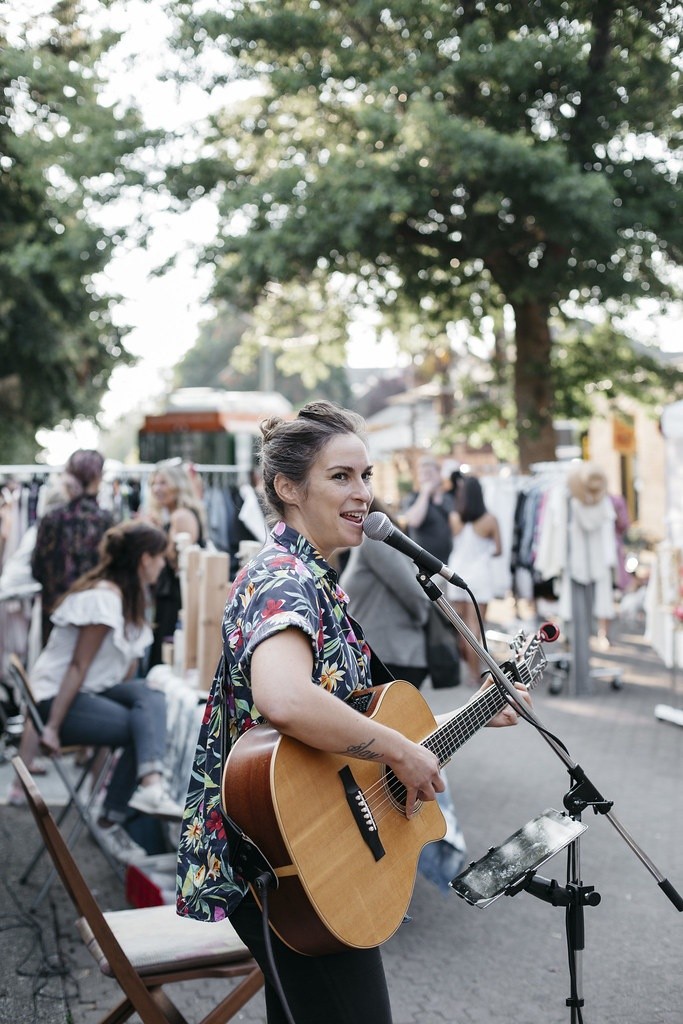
[12,753,267,1023]
[6,652,131,913]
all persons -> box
[29,518,183,829]
[340,537,431,690]
[553,471,631,651]
[147,457,205,670]
[445,476,502,684]
[403,460,451,566]
[175,401,534,1023]
[32,448,116,648]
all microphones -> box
[363,511,468,589]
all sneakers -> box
[126,779,185,822]
[90,814,147,865]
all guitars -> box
[220,622,561,957]
